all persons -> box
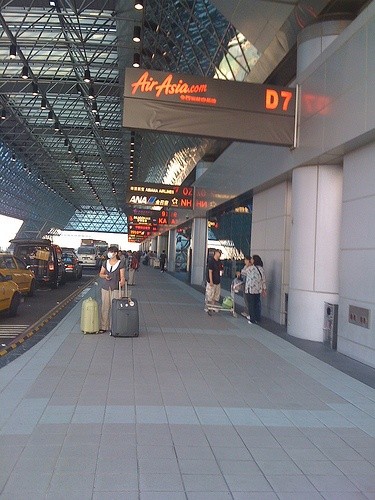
[99,249,157,267]
[241,255,268,325]
[98,254,105,273]
[127,250,136,285]
[236,255,254,320]
[98,246,126,336]
[204,249,224,317]
[159,250,166,273]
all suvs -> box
[0,239,108,317]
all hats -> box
[214,249,223,254]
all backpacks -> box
[130,254,139,270]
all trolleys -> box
[204,277,244,318]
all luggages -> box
[81,281,100,335]
[109,279,139,338]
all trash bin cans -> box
[323,301,339,350]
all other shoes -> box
[241,312,258,324]
[99,329,106,333]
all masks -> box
[108,252,114,259]
